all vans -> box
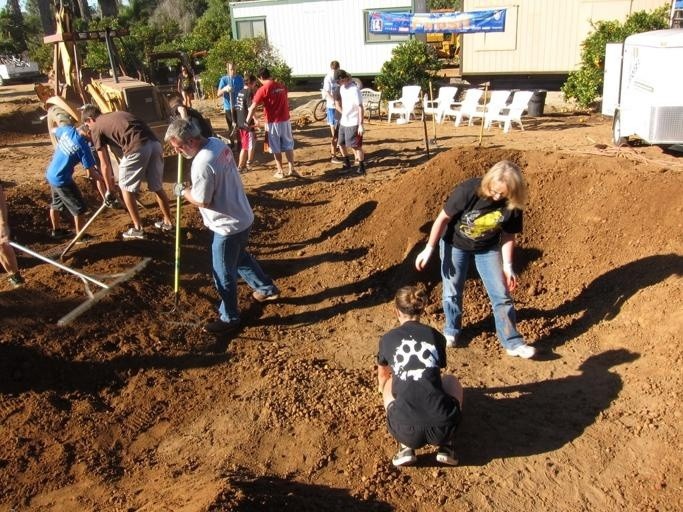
[144,47,195,96]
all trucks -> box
[0,38,42,85]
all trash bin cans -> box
[528,88,547,117]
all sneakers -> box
[330,155,365,177]
[273,170,284,179]
[122,227,145,240]
[50,228,76,241]
[249,290,281,303]
[435,443,460,467]
[443,334,459,348]
[288,169,299,176]
[154,221,173,230]
[505,342,537,359]
[76,233,96,243]
[392,446,417,467]
[7,272,25,288]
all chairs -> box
[439,87,484,127]
[469,89,511,129]
[358,87,381,123]
[386,84,423,124]
[420,85,457,124]
[484,91,534,133]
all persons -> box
[415,159,537,359]
[337,70,364,177]
[331,72,352,164]
[322,61,344,149]
[377,282,463,466]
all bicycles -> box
[312,87,328,123]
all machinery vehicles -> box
[33,0,195,170]
[425,5,461,65]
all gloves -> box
[414,242,435,273]
[502,262,517,293]
[173,182,186,197]
[358,124,365,137]
[222,85,233,93]
